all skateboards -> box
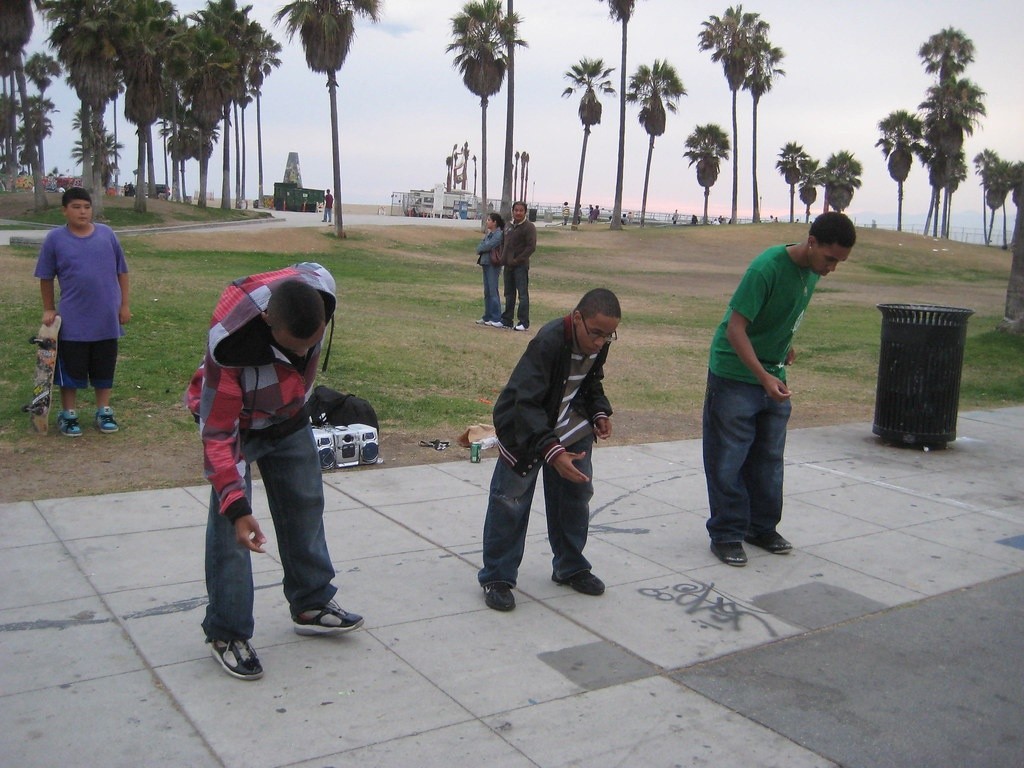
[21,314,62,435]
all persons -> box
[491,201,537,331]
[475,213,502,326]
[183,262,364,680]
[124,182,136,197]
[770,215,813,224]
[562,201,633,226]
[672,209,731,225]
[476,288,622,612]
[321,189,333,222]
[703,212,857,567]
[35,187,130,435]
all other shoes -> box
[322,219,327,222]
[484,320,492,325]
[475,319,484,324]
[328,220,331,221]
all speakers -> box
[347,424,379,464]
[311,428,335,470]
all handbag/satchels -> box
[304,385,380,433]
[490,231,502,267]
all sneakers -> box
[57,409,83,437]
[711,540,748,566]
[483,583,516,611]
[744,530,793,554]
[491,322,513,330]
[211,637,263,680]
[552,571,605,596]
[291,599,365,634]
[95,406,119,433]
[514,325,528,331]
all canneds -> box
[470,442,481,463]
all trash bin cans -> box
[872,302,975,451]
[545,209,553,223]
[186,196,193,203]
[529,209,538,222]
[253,200,259,208]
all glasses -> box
[582,319,617,343]
